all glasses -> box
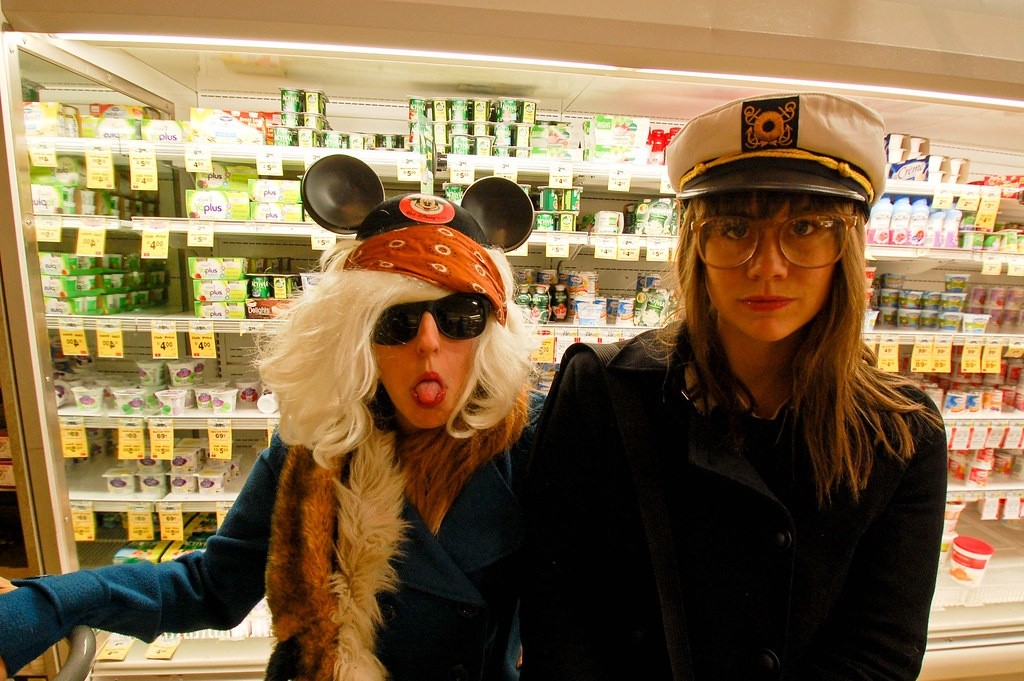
[370,294,494,345]
[688,213,859,268]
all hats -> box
[663,90,887,224]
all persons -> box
[0,156,557,681]
[516,91,948,681]
